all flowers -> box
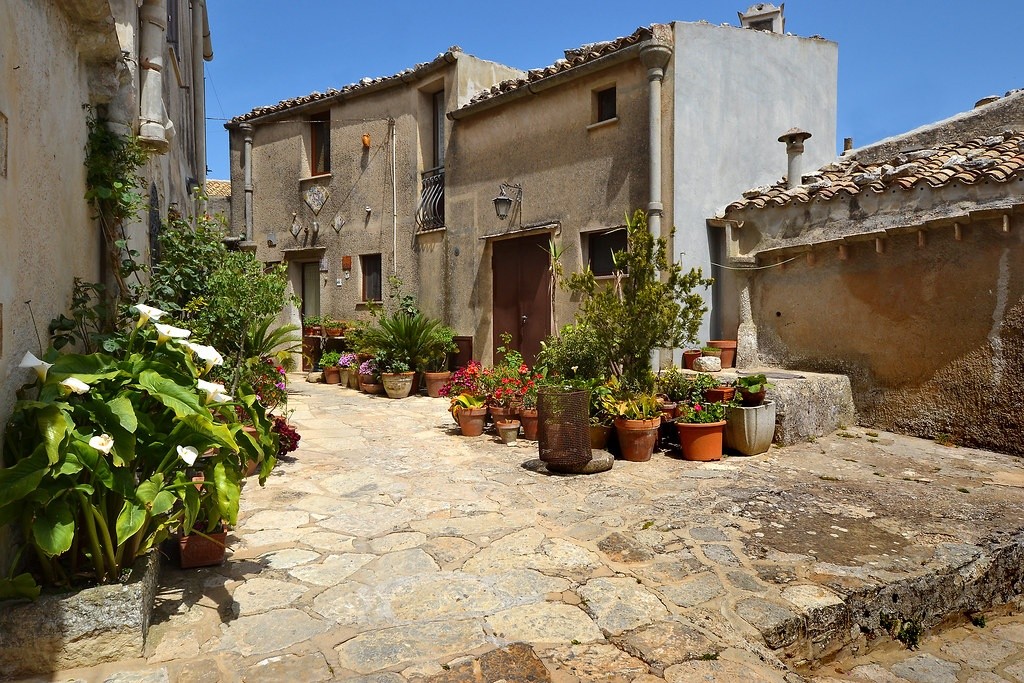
[676,402,723,423]
[220,360,287,424]
[357,359,380,374]
[438,361,542,406]
[337,352,357,368]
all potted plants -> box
[0,210,486,680]
[543,209,776,462]
[497,406,519,443]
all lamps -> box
[492,182,522,220]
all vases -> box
[339,367,349,386]
[706,340,736,368]
[522,409,539,441]
[674,420,727,461]
[363,373,380,383]
[683,350,701,369]
[487,406,519,435]
[235,426,263,472]
